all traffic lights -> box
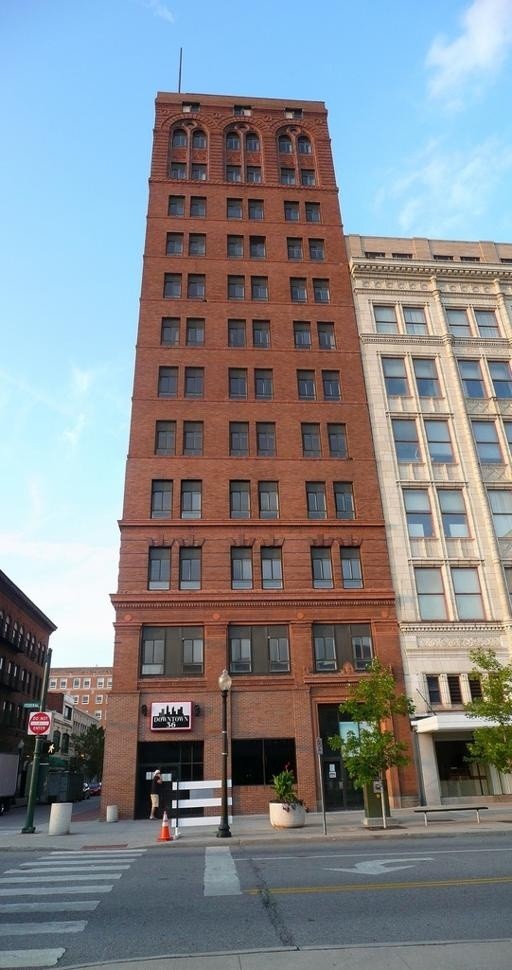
[43,742,57,755]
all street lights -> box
[216,668,231,837]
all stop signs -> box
[27,710,52,735]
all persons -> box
[149,769,164,820]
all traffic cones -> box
[156,812,171,841]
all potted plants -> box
[268,762,309,828]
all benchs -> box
[413,806,488,826]
